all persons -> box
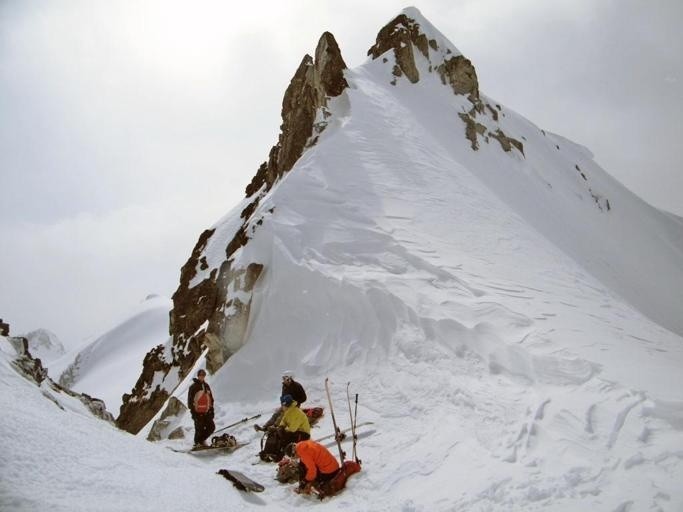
[285,441,339,495]
[283,371,307,408]
[262,395,286,432]
[259,395,310,462]
[188,370,215,449]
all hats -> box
[282,371,293,378]
[281,395,292,405]
[284,443,292,457]
